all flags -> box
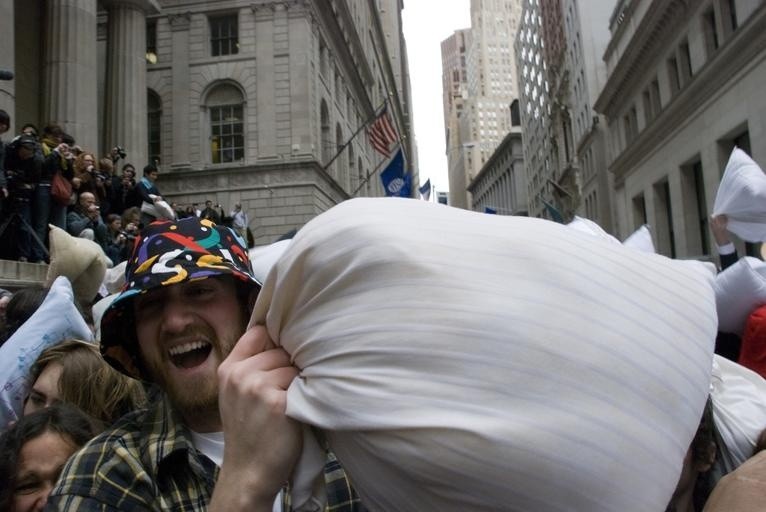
[380,147,410,198]
[404,172,413,198]
[541,202,564,223]
[365,101,398,159]
[485,207,497,214]
[417,179,431,200]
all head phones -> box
[14,139,38,154]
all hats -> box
[8,135,38,149]
[99,217,262,384]
[44,125,64,138]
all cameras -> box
[114,148,126,159]
[91,202,101,210]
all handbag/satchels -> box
[50,172,74,209]
[124,186,139,208]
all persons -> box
[0,110,363,512]
[666,212,765,512]
